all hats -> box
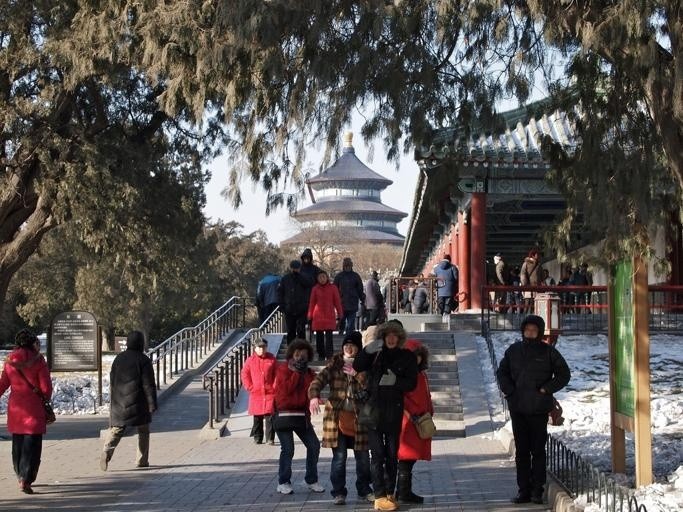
[343,331,362,350]
[342,257,352,267]
[254,338,267,347]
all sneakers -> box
[276,482,292,494]
[18,479,33,494]
[529,495,543,504]
[300,479,325,493]
[356,493,375,504]
[332,494,346,504]
[373,495,400,510]
[510,491,529,503]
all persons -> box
[397,338,437,502]
[364,270,384,326]
[354,320,419,510]
[300,248,322,283]
[434,254,459,315]
[382,276,431,315]
[497,314,572,504]
[243,338,276,445]
[333,258,365,333]
[0,328,53,493]
[520,249,545,313]
[308,332,376,503]
[486,252,519,313]
[271,338,327,493]
[559,263,592,312]
[308,271,343,360]
[101,331,157,471]
[278,260,310,346]
[544,268,556,286]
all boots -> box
[395,473,423,503]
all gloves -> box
[364,339,383,353]
[308,397,319,417]
[343,362,356,378]
[379,369,396,387]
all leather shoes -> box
[101,450,109,472]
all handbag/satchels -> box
[16,365,55,424]
[404,410,436,437]
[273,397,309,432]
[338,410,358,436]
